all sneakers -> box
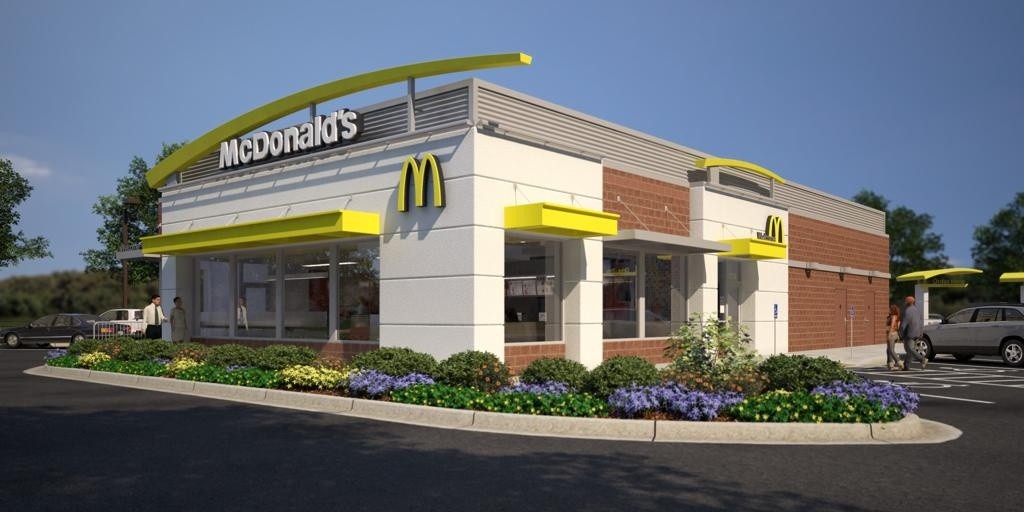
[920,356,929,370]
[887,358,911,371]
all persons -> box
[170,297,188,345]
[235,297,249,338]
[901,296,929,371]
[143,294,168,342]
[885,304,904,371]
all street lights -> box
[122,196,141,309]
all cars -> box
[0,312,133,350]
[97,308,149,338]
[927,312,944,326]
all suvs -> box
[602,306,671,338]
[912,303,1023,368]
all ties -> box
[155,307,158,328]
